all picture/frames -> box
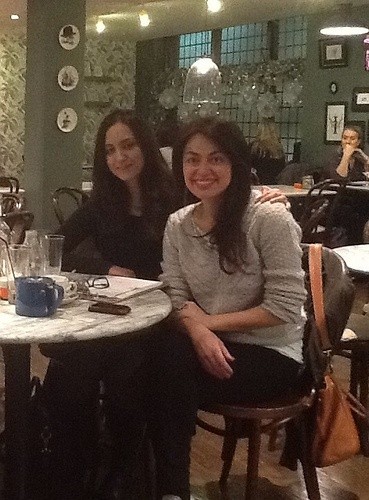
[346,120,366,150]
[350,86,369,113]
[324,102,347,145]
[318,36,350,67]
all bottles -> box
[23,229,43,276]
[303,176,313,188]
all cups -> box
[14,276,64,317]
[49,276,78,299]
[40,233,65,275]
[6,243,31,304]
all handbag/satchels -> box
[280,371,361,469]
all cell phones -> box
[89,302,130,314]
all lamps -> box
[319,0,369,35]
[182,1,227,102]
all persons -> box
[83,117,308,500]
[316,124,369,245]
[24,109,188,470]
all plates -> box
[60,294,78,306]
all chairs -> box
[0,176,87,258]
[194,164,369,500]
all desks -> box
[331,244,369,278]
[329,182,369,244]
[0,271,173,500]
[0,187,26,207]
[266,184,337,218]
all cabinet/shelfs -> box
[83,74,114,107]
